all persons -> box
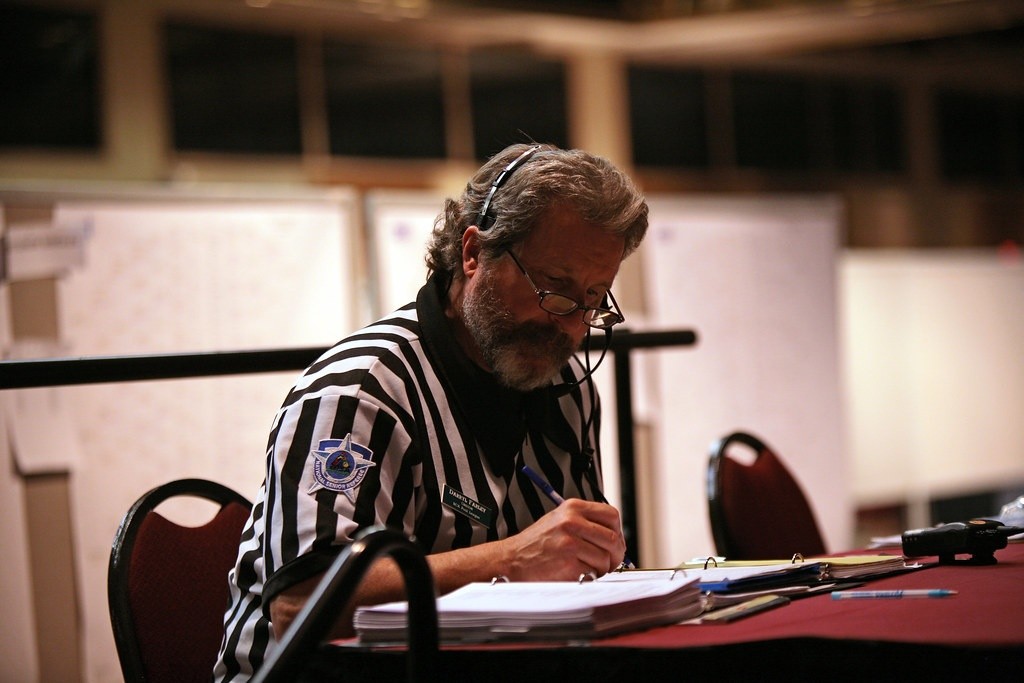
[205,144,649,683]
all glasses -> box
[504,249,625,329]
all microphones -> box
[543,334,608,399]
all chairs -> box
[707,431,827,563]
[107,478,253,683]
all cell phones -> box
[900,515,1024,559]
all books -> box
[353,548,913,642]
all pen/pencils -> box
[521,465,636,570]
[832,589,959,599]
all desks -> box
[323,539,1024,683]
